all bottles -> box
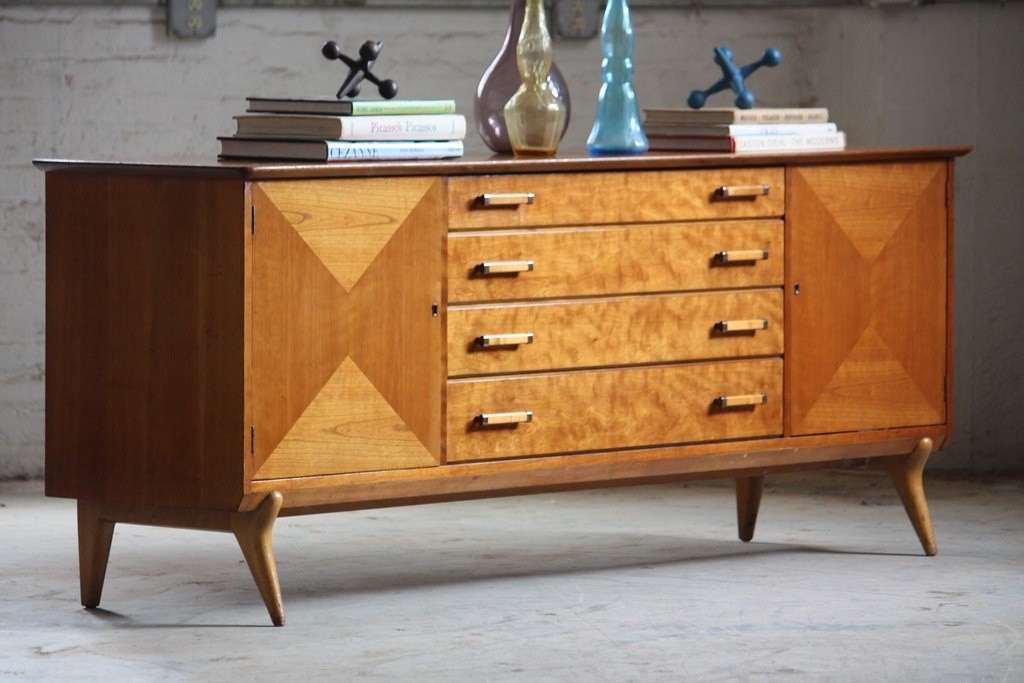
[476,0,570,156]
[504,0,566,159]
[586,0,650,157]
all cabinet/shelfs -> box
[31,146,973,626]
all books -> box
[640,105,847,154]
[215,96,468,163]
